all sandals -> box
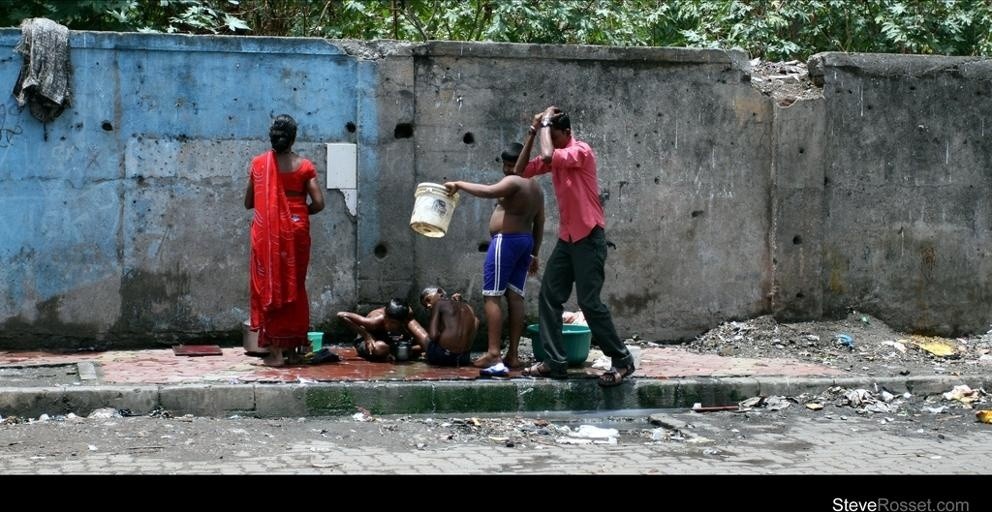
[521,362,569,379]
[597,359,635,387]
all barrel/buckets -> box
[409,182,459,238]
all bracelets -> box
[530,254,540,261]
[527,124,538,135]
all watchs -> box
[540,119,553,127]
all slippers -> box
[478,362,510,376]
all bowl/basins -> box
[527,323,592,366]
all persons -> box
[332,297,413,361]
[243,112,327,369]
[509,104,637,388]
[443,140,547,370]
[408,284,482,368]
